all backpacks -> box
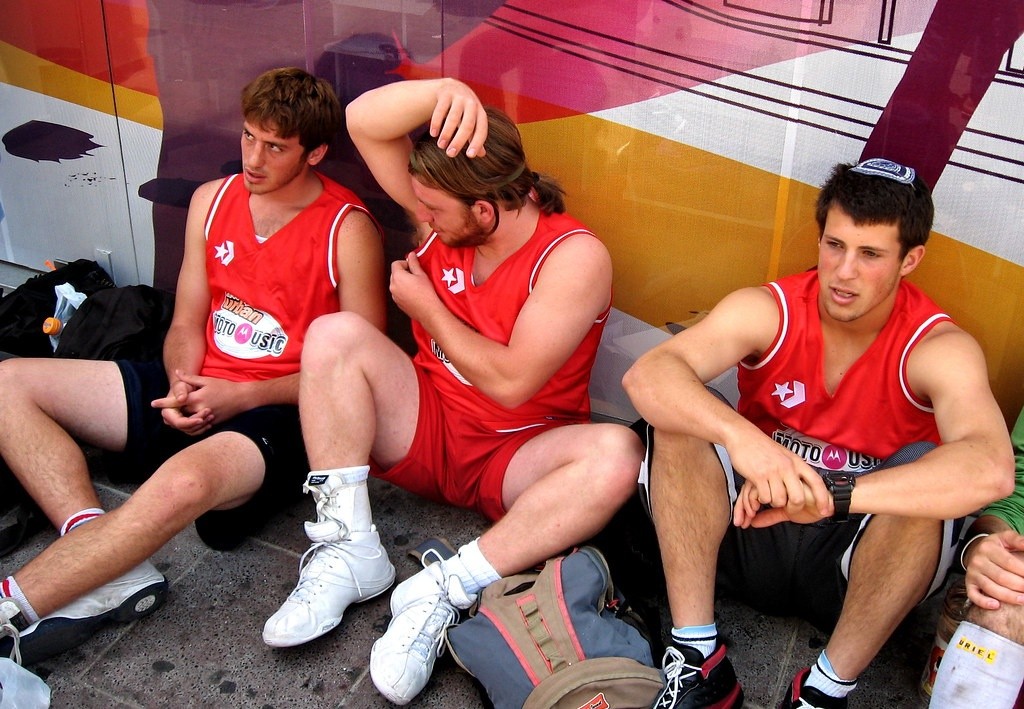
[446,546,664,709]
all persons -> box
[926,407,1024,709]
[260,77,646,705]
[621,157,1015,709]
[0,64,390,664]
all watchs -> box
[815,470,855,530]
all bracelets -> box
[961,533,991,573]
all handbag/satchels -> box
[1,259,125,358]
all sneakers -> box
[781,666,849,709]
[649,637,743,709]
[0,560,167,666]
[369,548,479,705]
[261,473,396,646]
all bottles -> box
[43,316,69,344]
[919,578,972,707]
